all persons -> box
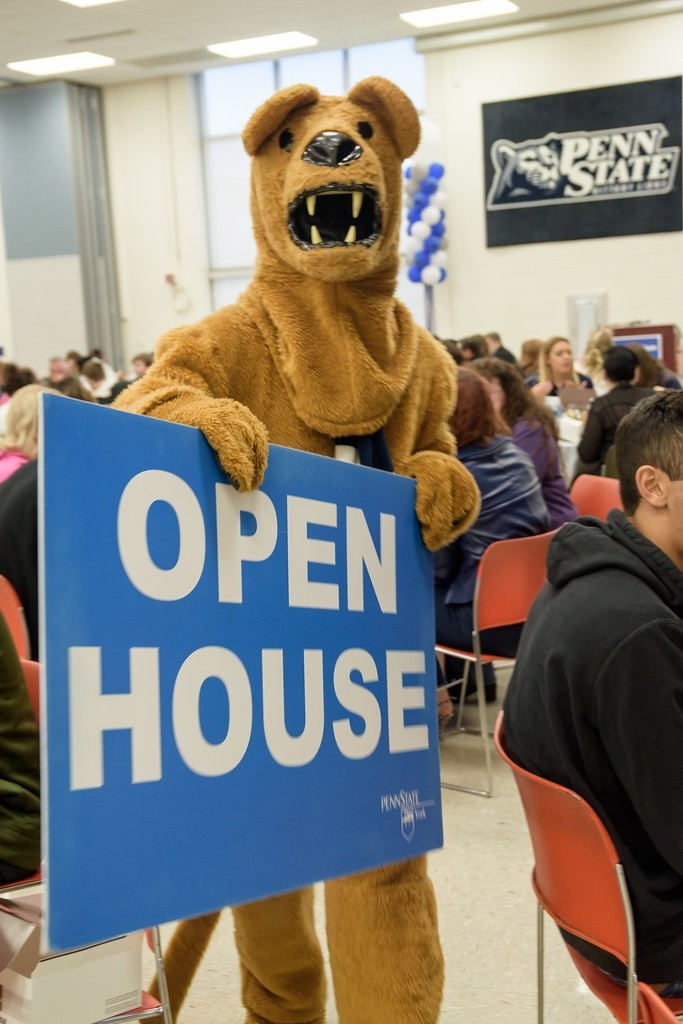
[0,326,682,1009]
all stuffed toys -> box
[108,75,482,1024]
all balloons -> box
[401,162,450,287]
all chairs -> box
[570,474,627,525]
[434,522,577,798]
[495,710,683,1024]
[0,658,172,1024]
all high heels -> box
[449,679,497,702]
[438,698,454,741]
